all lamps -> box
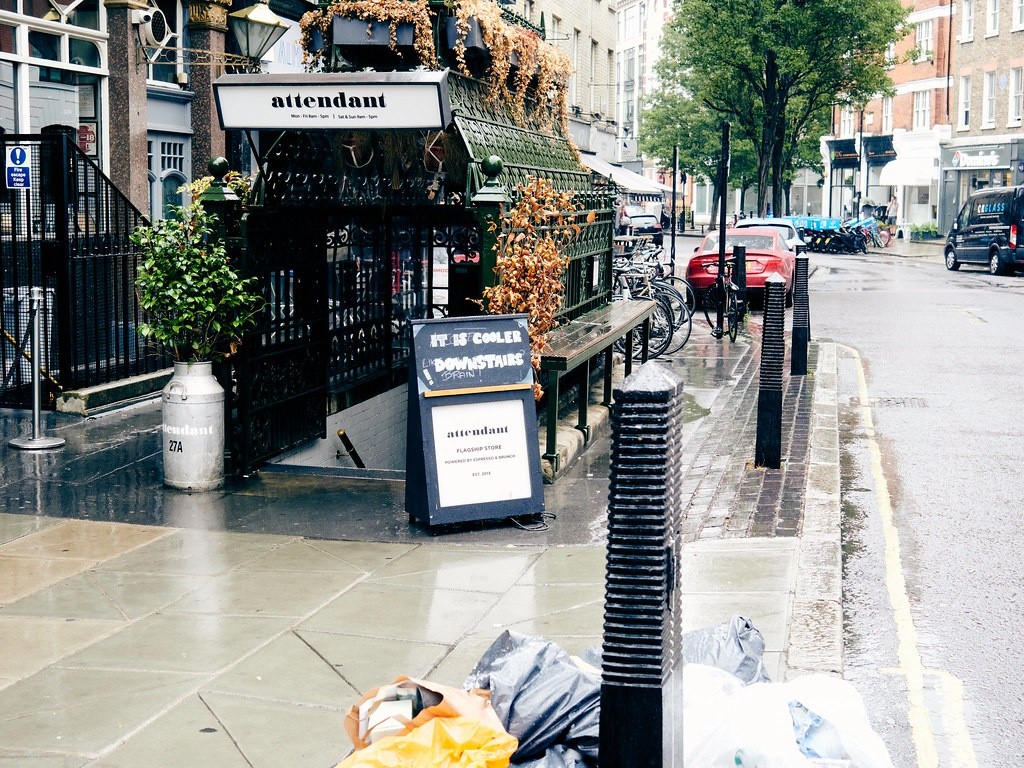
[606,120,617,126]
[571,106,582,118]
[137,0,292,74]
[622,127,629,132]
[590,113,601,122]
[177,72,190,89]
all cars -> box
[685,227,795,308]
[733,218,801,251]
[614,215,663,247]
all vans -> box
[947,185,1024,276]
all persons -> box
[886,195,899,237]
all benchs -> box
[541,298,656,472]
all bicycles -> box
[701,256,746,344]
[802,221,885,254]
[612,244,701,360]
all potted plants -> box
[128,204,272,491]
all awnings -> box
[577,152,683,202]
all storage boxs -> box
[782,215,841,230]
